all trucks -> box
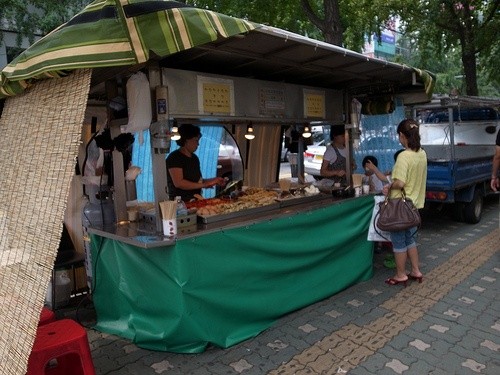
[349,93,500,225]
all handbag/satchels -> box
[376,182,421,231]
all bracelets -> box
[491,176,497,180]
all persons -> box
[165,124,226,202]
[320,124,356,184]
[491,128,500,192]
[381,119,428,286]
[363,149,405,251]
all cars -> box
[280,135,298,163]
[169,127,243,196]
[302,132,404,181]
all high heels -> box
[384,276,409,288]
[406,273,423,283]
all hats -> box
[179,124,200,137]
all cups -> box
[353,184,363,196]
[362,185,370,193]
[162,219,177,237]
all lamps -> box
[301,124,311,138]
[245,120,255,140]
[170,119,181,141]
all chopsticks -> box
[351,173,363,188]
[159,200,177,220]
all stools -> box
[25,306,95,375]
